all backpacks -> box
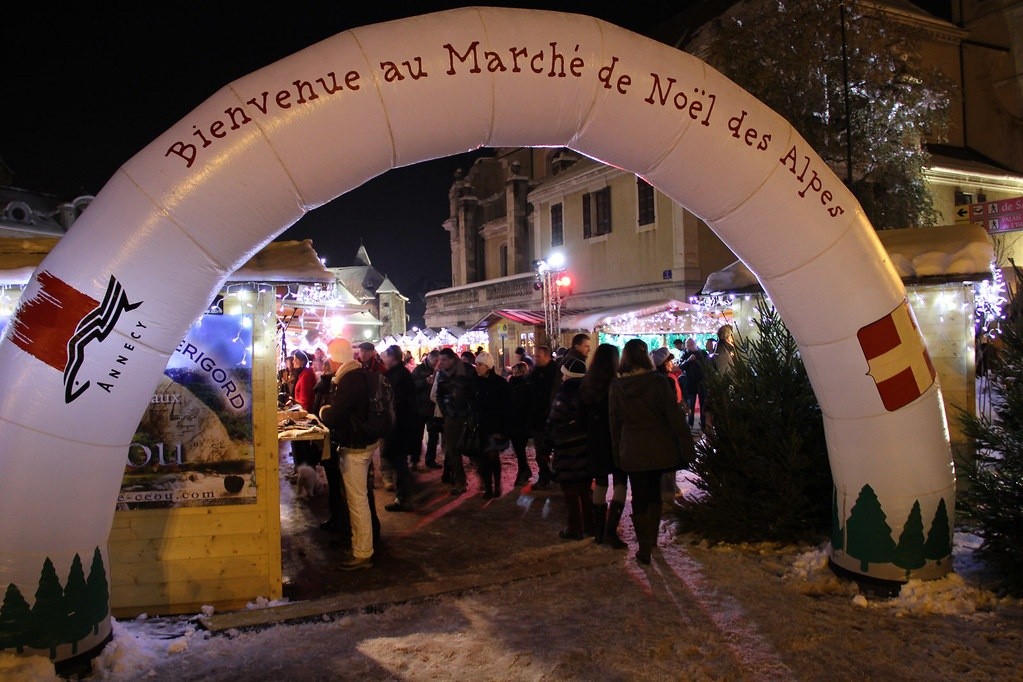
[350,367,396,439]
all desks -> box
[278,406,325,442]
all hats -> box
[517,361,528,377]
[561,357,588,378]
[476,351,495,370]
[327,338,354,363]
[652,347,670,366]
[358,343,375,351]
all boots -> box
[630,510,662,565]
[591,500,628,549]
[559,500,584,541]
[581,492,594,534]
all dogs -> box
[296,465,322,497]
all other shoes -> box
[532,472,550,490]
[514,469,532,486]
[385,500,413,512]
[426,461,443,469]
[319,517,343,534]
[384,481,395,491]
[483,488,501,499]
[451,484,467,495]
[412,494,423,504]
[412,465,422,472]
[338,556,373,570]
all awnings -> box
[276,282,383,331]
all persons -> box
[278,325,732,572]
